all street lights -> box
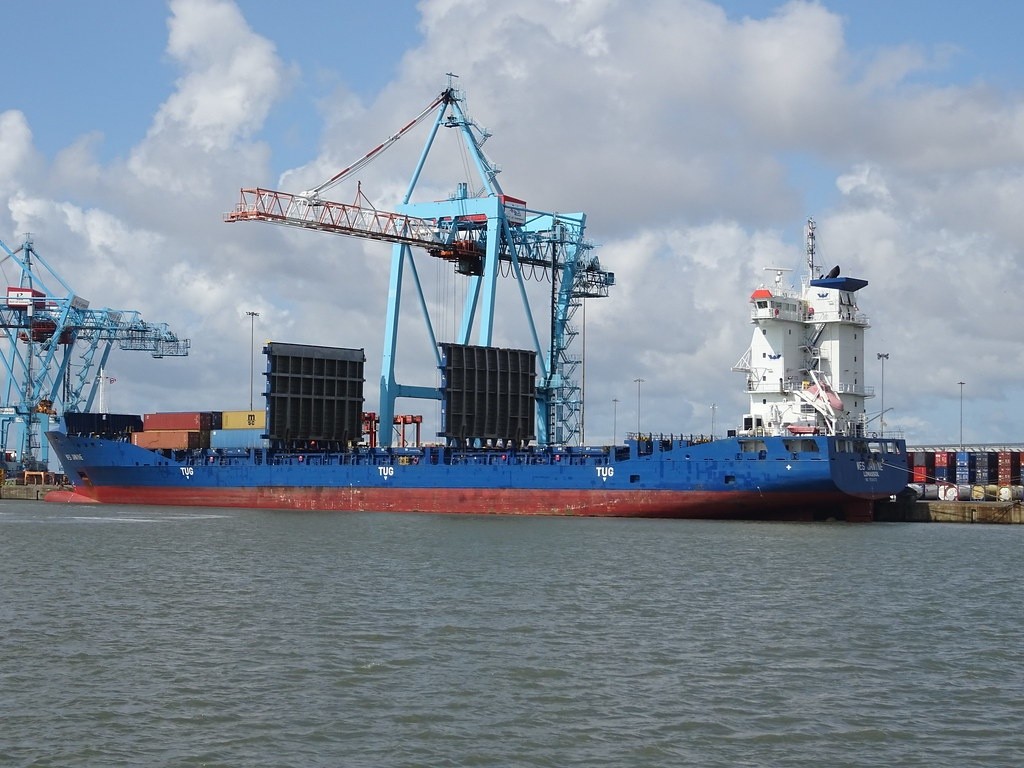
[709,402,719,442]
[96,366,110,414]
[957,381,967,452]
[633,377,645,437]
[876,353,889,436]
[611,398,619,445]
[245,310,260,411]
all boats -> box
[42,215,912,522]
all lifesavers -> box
[413,458,419,464]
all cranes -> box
[1,230,191,477]
[220,71,619,448]
